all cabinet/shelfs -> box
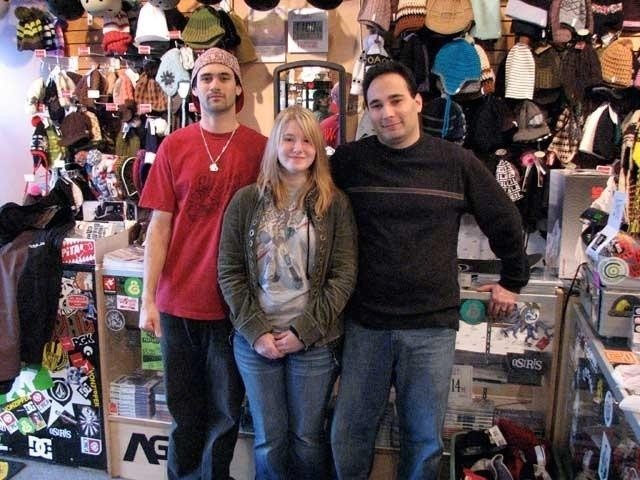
[0,227,107,472]
[551,294,640,480]
[93,229,561,480]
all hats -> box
[14,0,259,64]
[494,0,639,234]
[31,46,244,198]
[351,1,518,157]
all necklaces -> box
[199,123,240,173]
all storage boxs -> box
[447,429,566,480]
[577,260,639,341]
[544,167,613,280]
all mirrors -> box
[272,59,346,160]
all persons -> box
[309,81,340,149]
[136,47,266,480]
[329,62,530,480]
[215,106,358,480]
